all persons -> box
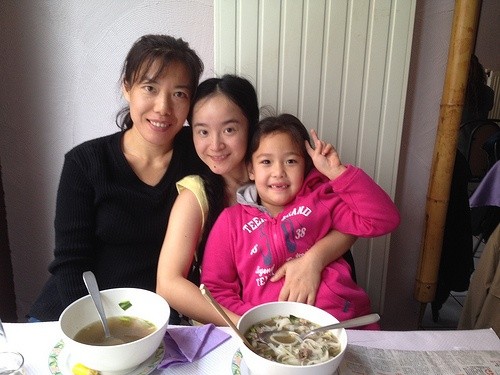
[155,75,360,328]
[26,34,205,323]
[200,113,401,331]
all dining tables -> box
[0,322,500,375]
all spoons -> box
[258,313,381,348]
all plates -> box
[48,338,166,375]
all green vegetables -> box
[119,301,154,326]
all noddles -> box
[246,314,341,366]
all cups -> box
[0,350,25,375]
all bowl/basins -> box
[237,301,348,375]
[58,288,171,375]
